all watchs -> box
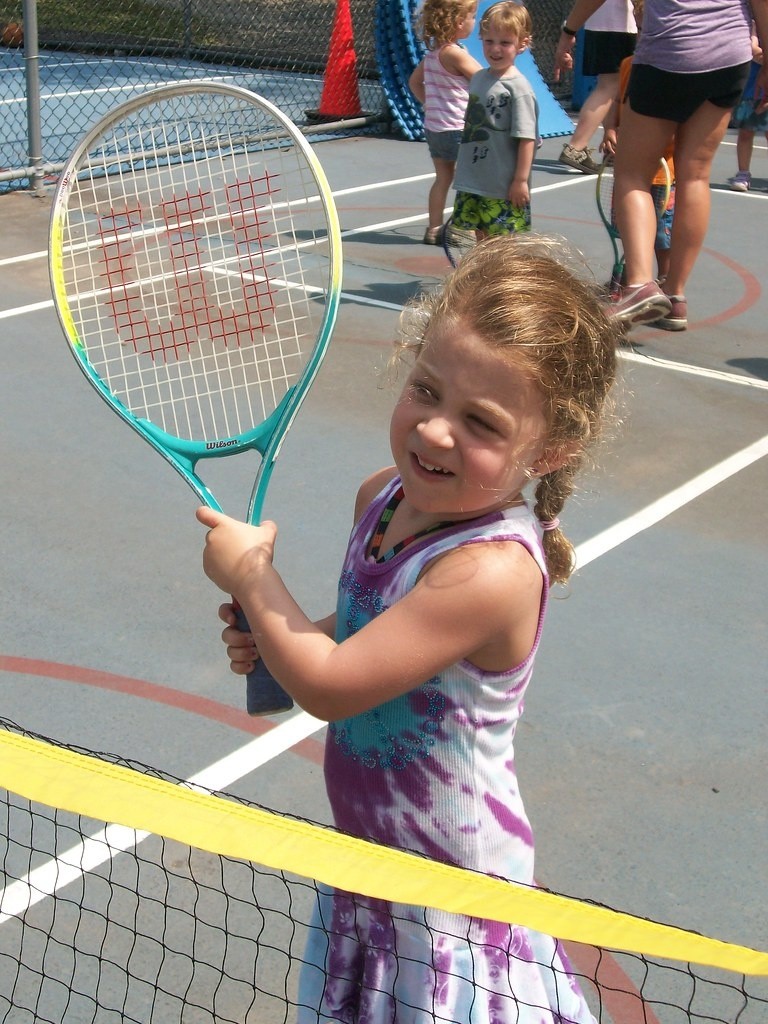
[561,20,576,37]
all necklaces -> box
[367,483,462,565]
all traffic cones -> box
[304,0,378,123]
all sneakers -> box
[559,142,604,174]
[729,170,752,191]
[424,223,446,245]
[602,280,674,332]
[654,294,690,331]
[603,146,615,167]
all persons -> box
[409,0,768,331]
[197,231,618,1024]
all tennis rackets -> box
[443,213,478,268]
[595,147,671,302]
[45,78,345,718]
